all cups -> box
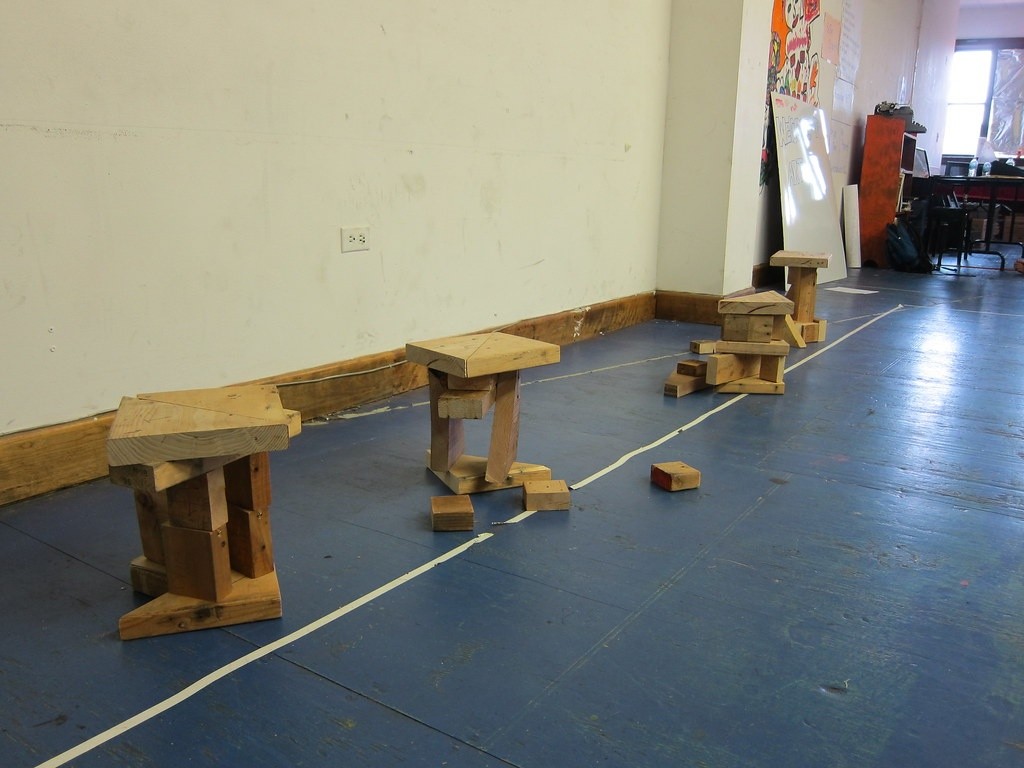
[982,161,991,176]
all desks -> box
[912,175,1024,270]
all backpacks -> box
[886,217,934,273]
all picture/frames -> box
[916,147,930,178]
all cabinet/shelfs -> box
[856,114,918,259]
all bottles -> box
[968,157,978,177]
[1006,157,1014,166]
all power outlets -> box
[341,227,370,253]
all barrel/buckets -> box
[971,218,987,248]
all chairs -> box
[923,177,981,267]
